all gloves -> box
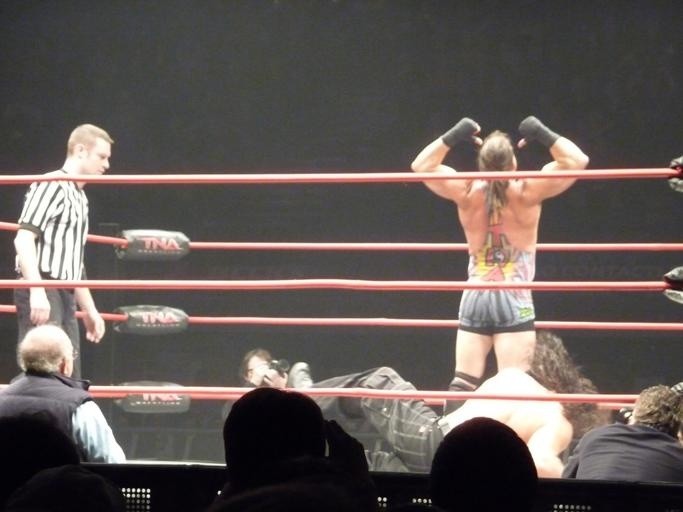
[518,115,563,152]
[441,117,478,149]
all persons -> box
[561,385,683,482]
[8,122,116,390]
[426,417,537,512]
[408,111,589,417]
[288,332,609,477]
[210,388,369,511]
[0,323,127,512]
[221,347,287,419]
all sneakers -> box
[286,359,314,388]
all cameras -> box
[260,358,291,387]
